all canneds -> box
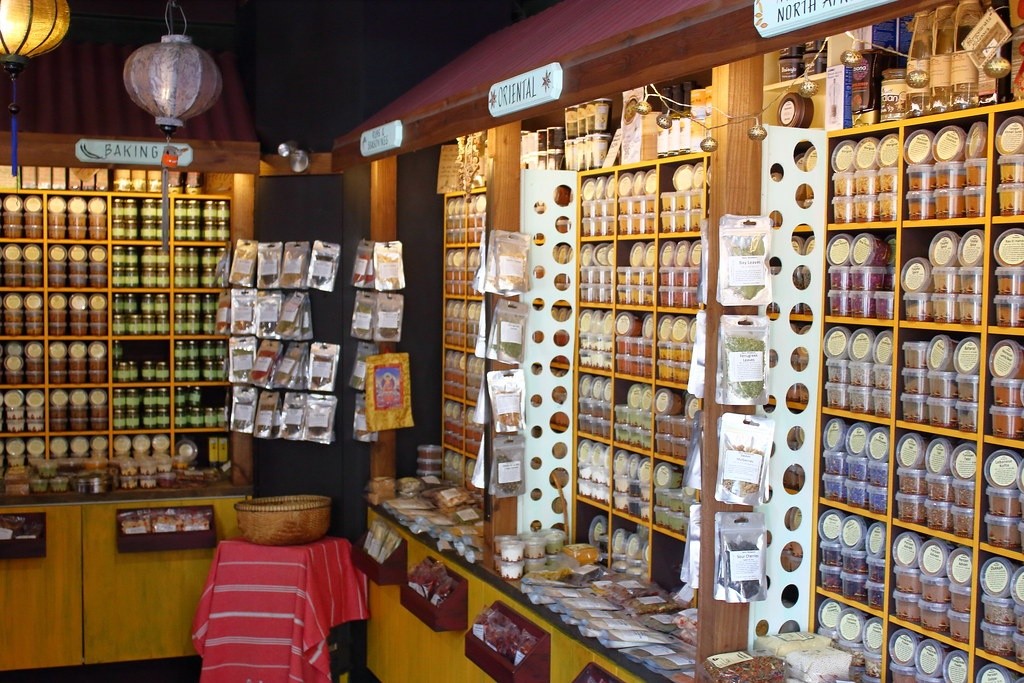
[880,69,910,122]
[112,198,230,428]
[536,97,614,171]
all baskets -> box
[233,494,332,546]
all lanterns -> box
[122,32,223,253]
[0,0,70,176]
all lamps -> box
[278,143,310,173]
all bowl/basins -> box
[0,198,198,494]
[418,119,1023,683]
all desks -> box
[191,533,369,683]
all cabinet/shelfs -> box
[0,99,1024,683]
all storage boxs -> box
[572,661,631,683]
[851,53,899,115]
[398,555,471,633]
[347,521,410,585]
[466,599,553,683]
[0,512,47,560]
[853,16,915,53]
[825,63,853,131]
[640,111,662,161]
[114,505,217,553]
[436,143,484,195]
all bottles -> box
[113,197,230,429]
